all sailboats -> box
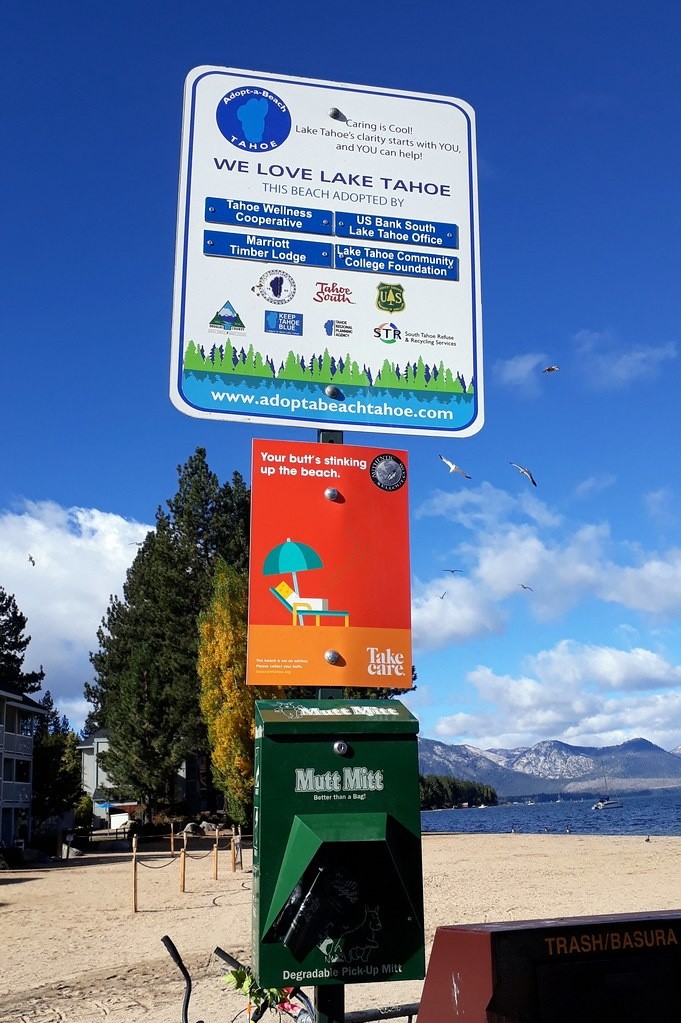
[590,750,625,811]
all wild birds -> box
[543,365,560,375]
[28,554,35,567]
[544,827,549,834]
[436,592,446,599]
[511,826,515,834]
[442,570,463,573]
[518,584,534,592]
[508,461,537,487]
[566,826,570,834]
[439,454,472,479]
[645,836,650,842]
